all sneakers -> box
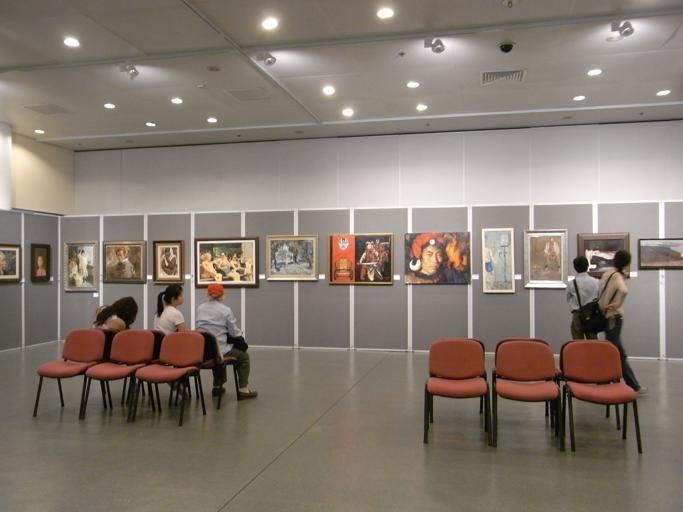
[636,386,648,395]
[238,390,257,400]
[211,387,226,396]
[166,381,188,399]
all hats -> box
[206,282,225,299]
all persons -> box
[77,249,88,279]
[108,246,135,278]
[596,250,649,395]
[36,255,46,276]
[94,296,138,334]
[566,255,600,340]
[405,233,448,284]
[194,283,258,399]
[153,283,185,397]
[201,251,253,282]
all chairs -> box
[27,326,242,428]
[416,337,646,455]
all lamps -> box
[421,33,447,55]
[250,48,279,69]
[605,14,635,38]
[116,61,141,81]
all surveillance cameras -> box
[497,41,515,52]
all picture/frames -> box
[264,233,318,282]
[0,242,23,285]
[62,238,99,293]
[326,231,395,288]
[635,235,683,271]
[520,228,569,292]
[575,231,632,280]
[29,242,51,283]
[479,225,516,296]
[100,239,148,286]
[191,235,259,289]
[151,238,185,287]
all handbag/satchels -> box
[577,301,606,335]
